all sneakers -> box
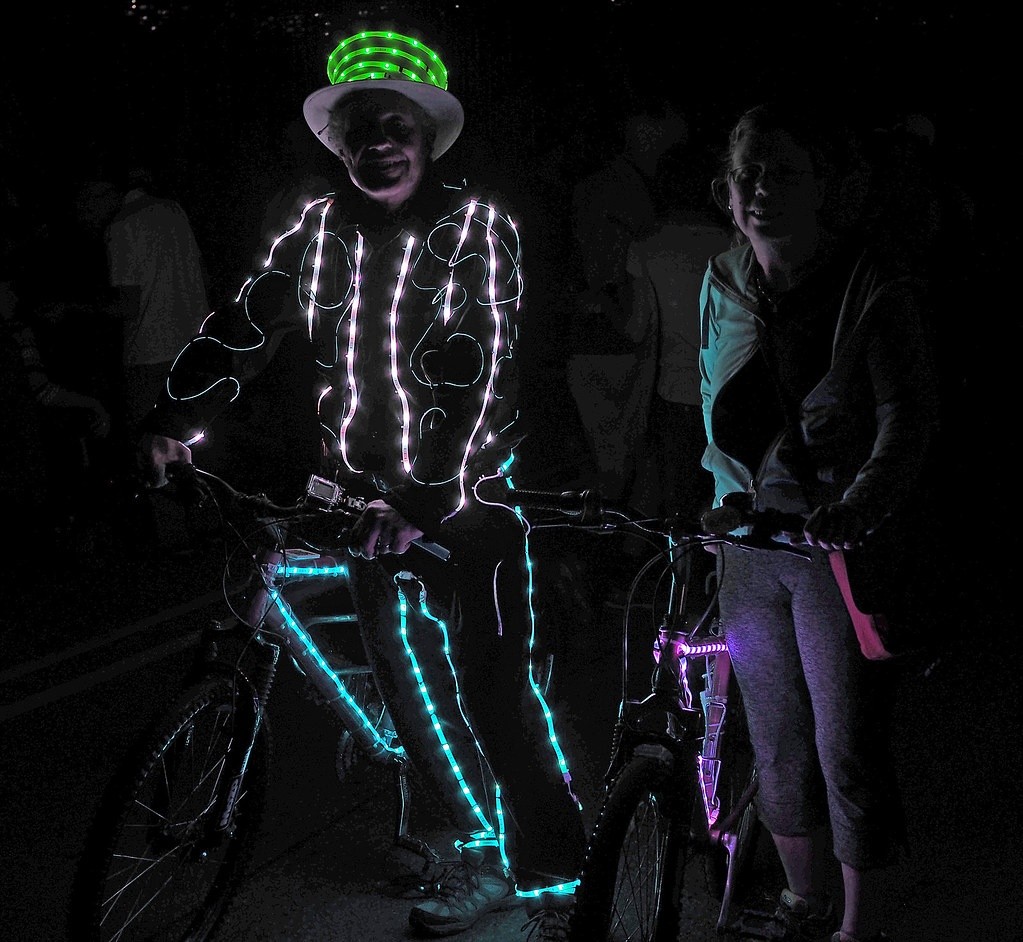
[519,891,582,942]
[408,859,518,935]
[739,888,837,942]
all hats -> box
[303,31,466,163]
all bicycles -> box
[68,459,554,942]
[506,486,788,942]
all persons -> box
[0,28,1022,942]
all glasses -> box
[728,161,819,186]
